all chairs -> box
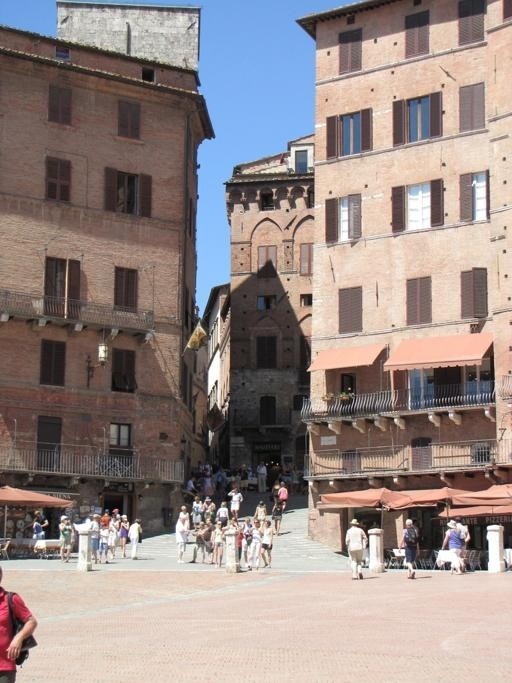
[385,548,483,572]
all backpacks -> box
[406,527,417,544]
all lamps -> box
[85,342,109,388]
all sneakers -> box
[352,573,363,580]
[408,571,415,579]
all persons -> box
[440,517,471,575]
[32,518,42,539]
[345,519,368,580]
[23,511,49,538]
[399,518,420,579]
[57,509,142,564]
[0,567,38,683]
[174,458,302,572]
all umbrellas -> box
[0,486,75,543]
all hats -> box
[350,519,359,525]
[61,515,68,521]
[447,520,457,529]
[205,497,212,501]
[93,514,101,518]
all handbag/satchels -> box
[71,532,76,543]
[246,535,253,545]
[14,619,37,665]
[139,533,142,543]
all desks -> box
[35,539,60,559]
